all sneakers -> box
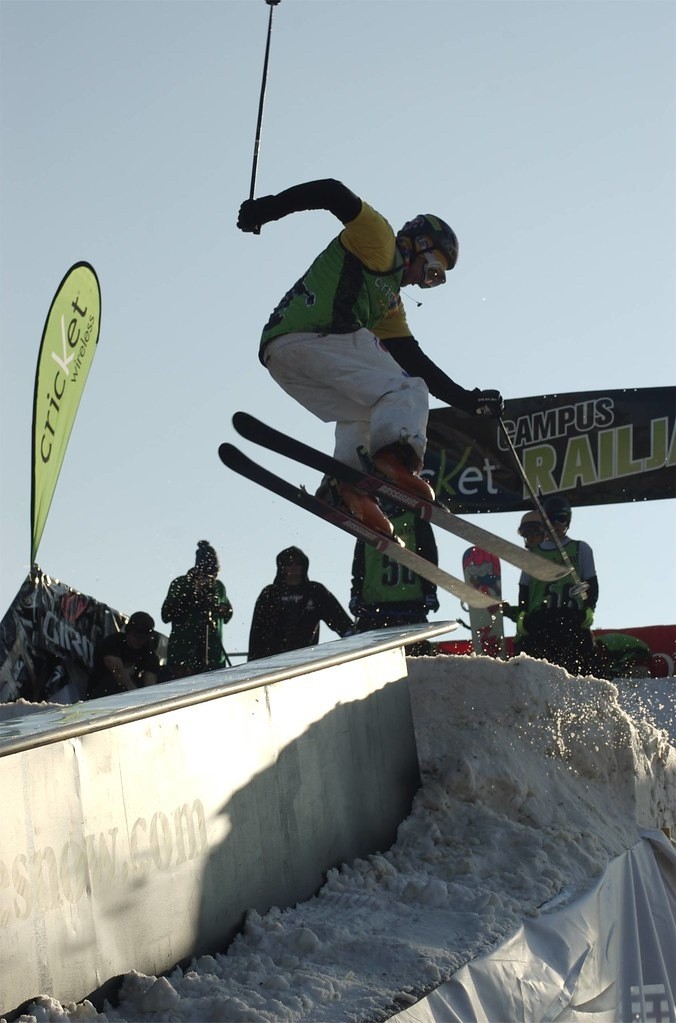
[327,476,395,539]
[355,444,436,503]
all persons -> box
[89,498,650,698]
[237,178,507,546]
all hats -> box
[125,612,154,633]
[277,547,309,585]
[196,540,217,566]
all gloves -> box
[501,601,517,623]
[349,589,368,617]
[237,195,279,232]
[455,388,506,418]
[517,611,529,636]
[583,606,594,628]
[424,589,439,614]
[220,603,232,624]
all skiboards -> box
[217,409,576,612]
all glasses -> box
[422,261,446,289]
[548,515,569,526]
[284,559,303,566]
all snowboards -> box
[462,544,508,661]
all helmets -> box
[543,496,571,529]
[397,214,458,270]
[518,511,543,539]
